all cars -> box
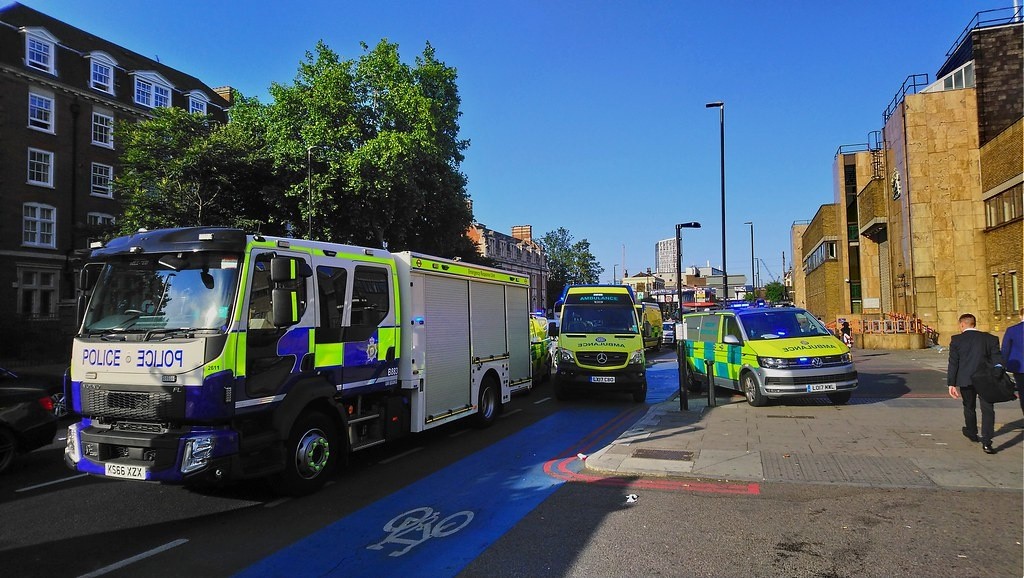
[0,361,72,474]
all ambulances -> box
[676,302,857,407]
[530,282,663,399]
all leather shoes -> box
[962,427,978,442]
[983,444,992,453]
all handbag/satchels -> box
[970,367,1018,403]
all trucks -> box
[61,226,531,490]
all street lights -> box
[614,264,620,284]
[744,220,756,303]
[898,275,908,317]
[675,220,702,410]
[307,146,330,241]
[789,291,795,305]
[845,279,853,314]
[706,100,732,306]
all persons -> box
[1000,307,1024,415]
[817,317,825,326]
[946,314,1002,453]
[841,322,850,343]
